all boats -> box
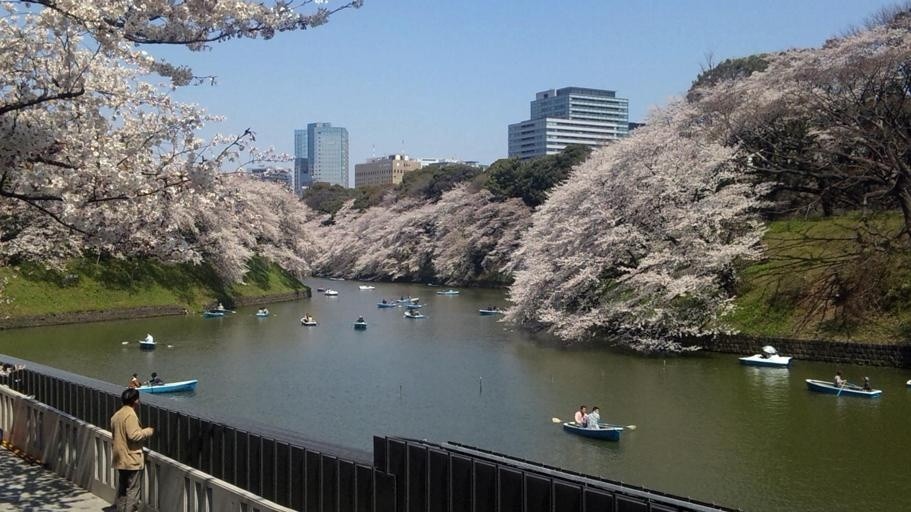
[255,312,268,317]
[479,309,509,315]
[202,310,224,318]
[300,318,316,326]
[353,321,366,330]
[737,355,792,368]
[138,340,157,349]
[805,379,882,399]
[125,379,198,394]
[562,422,623,442]
[325,284,460,318]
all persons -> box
[587,406,603,429]
[860,375,875,393]
[126,372,142,388]
[834,368,849,388]
[108,387,156,512]
[149,372,164,386]
[143,331,155,343]
[572,405,589,430]
[210,281,502,330]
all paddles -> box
[122,340,175,348]
[551,417,636,430]
